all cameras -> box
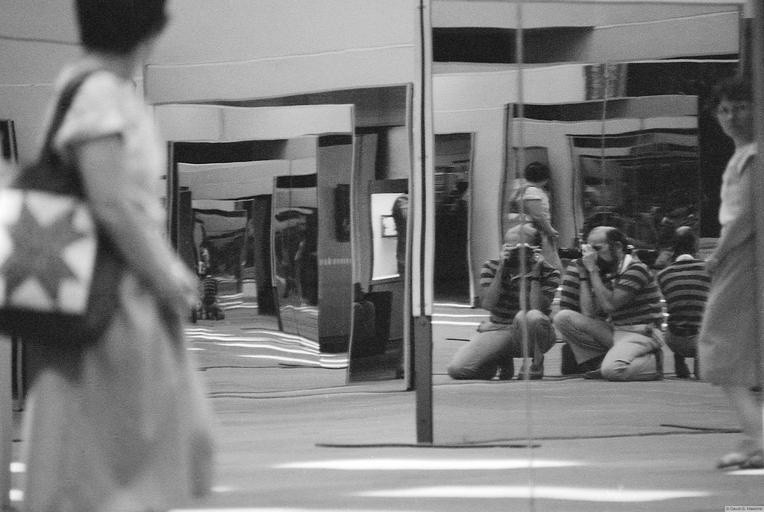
[507,243,535,258]
[558,246,584,259]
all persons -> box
[448,74,764,470]
[196,267,226,319]
[24,0,219,512]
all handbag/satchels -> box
[0,146,118,348]
